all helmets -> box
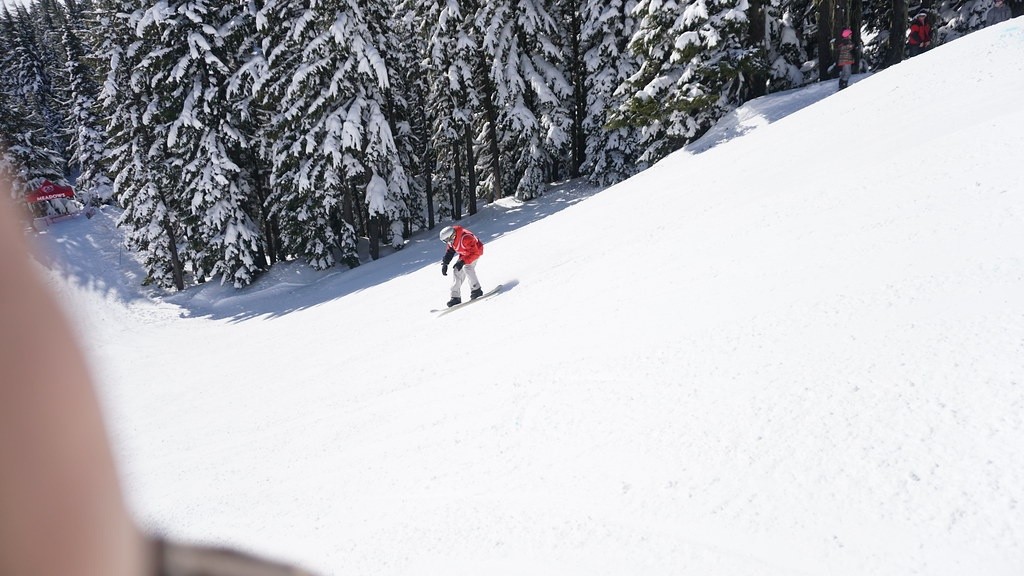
[439,226,457,244]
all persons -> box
[0,164,326,576]
[836,29,856,90]
[909,12,932,58]
[440,226,483,307]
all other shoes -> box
[470,287,483,300]
[447,297,461,307]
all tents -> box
[26,180,77,228]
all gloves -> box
[441,263,448,275]
[453,259,465,271]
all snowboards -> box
[430,285,503,313]
